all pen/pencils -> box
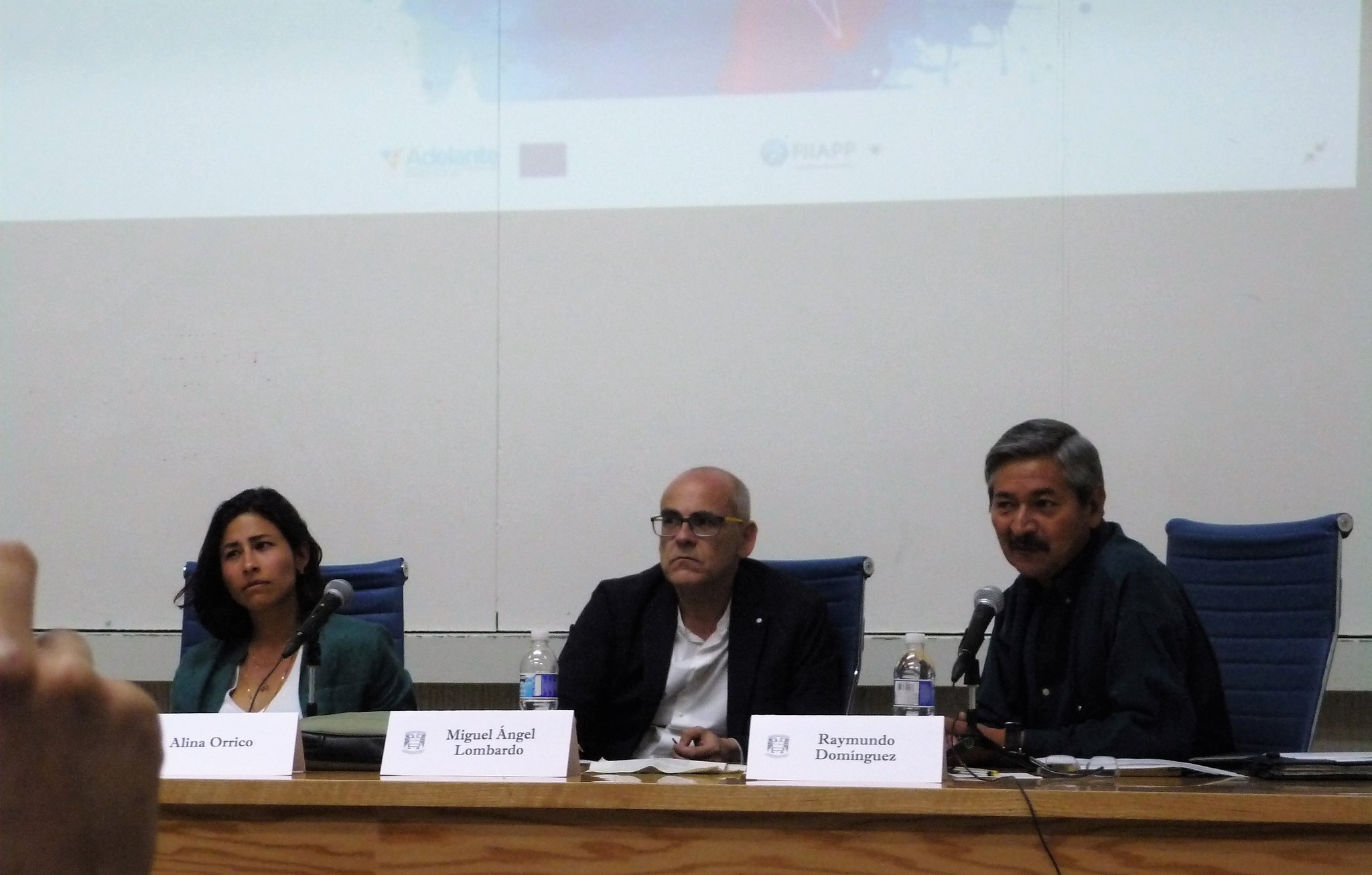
[948,769,998,777]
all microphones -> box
[279,577,354,660]
[951,584,1005,684]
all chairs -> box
[761,555,876,715]
[1165,513,1355,753]
[181,559,405,658]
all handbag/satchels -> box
[301,711,391,770]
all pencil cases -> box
[300,711,391,770]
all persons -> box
[556,466,830,765]
[939,418,1235,768]
[0,540,164,875]
[166,487,415,769]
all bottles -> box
[520,629,559,710]
[891,633,934,716]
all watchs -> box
[1002,721,1023,756]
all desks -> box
[152,762,1372,874]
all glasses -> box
[1024,754,1123,781]
[650,513,746,538]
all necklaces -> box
[243,650,294,713]
